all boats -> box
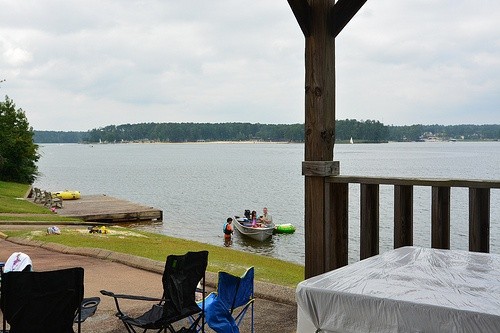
[231,216,275,242]
[51,189,81,200]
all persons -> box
[250,207,272,227]
[223,218,234,238]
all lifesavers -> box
[273,223,295,233]
[52,191,81,199]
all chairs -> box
[33,188,62,209]
[0,251,258,333]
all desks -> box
[295,246,500,333]
